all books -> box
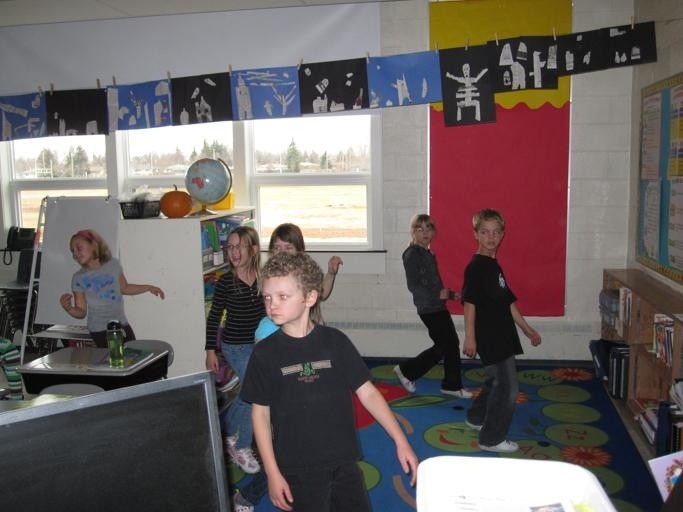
[608,286,683,503]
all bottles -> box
[106,321,124,368]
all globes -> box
[185,157,232,217]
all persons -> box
[233,224,342,512]
[461,208,542,452]
[394,214,472,398]
[60,229,164,347]
[206,225,266,473]
[239,252,418,512]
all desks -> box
[19,346,169,394]
[0,400,46,414]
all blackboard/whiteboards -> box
[35,196,120,326]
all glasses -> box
[226,243,249,249]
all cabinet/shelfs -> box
[592,268,634,411]
[629,290,682,512]
[118,205,261,377]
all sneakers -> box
[439,388,472,399]
[464,418,484,431]
[479,439,518,453]
[231,492,254,512]
[225,435,260,474]
[393,364,416,393]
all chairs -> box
[0,250,59,353]
[40,383,104,401]
[125,339,173,379]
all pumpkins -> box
[159,184,193,219]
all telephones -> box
[7,226,35,251]
